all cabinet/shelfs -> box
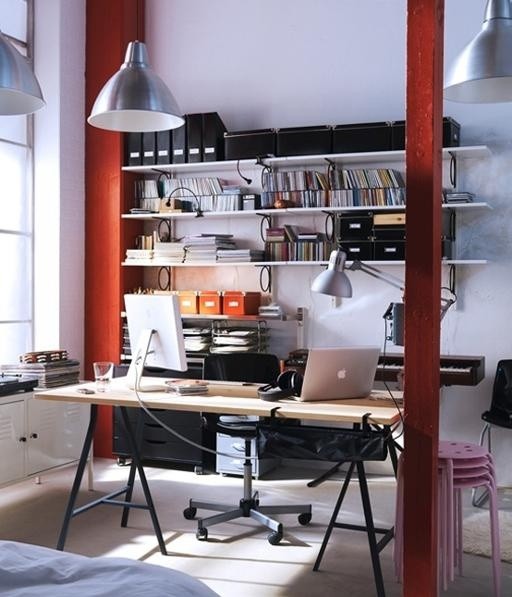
[121,143,495,267]
[0,379,94,492]
[118,309,301,363]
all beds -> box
[0,538,220,597]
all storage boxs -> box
[150,289,261,316]
[223,116,461,160]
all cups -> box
[92,360,115,392]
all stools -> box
[392,440,501,597]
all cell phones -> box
[77,389,95,394]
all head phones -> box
[257,369,303,401]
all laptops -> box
[286,346,381,402]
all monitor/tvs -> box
[123,293,188,392]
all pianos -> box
[284,349,484,385]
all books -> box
[121,223,332,262]
[129,178,248,215]
[441,190,474,203]
[1,358,79,390]
[260,169,406,210]
[120,325,270,354]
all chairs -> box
[471,358,512,506]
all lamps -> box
[442,0,512,103]
[310,250,454,347]
[0,0,186,131]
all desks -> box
[32,374,405,597]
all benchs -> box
[182,349,312,545]
